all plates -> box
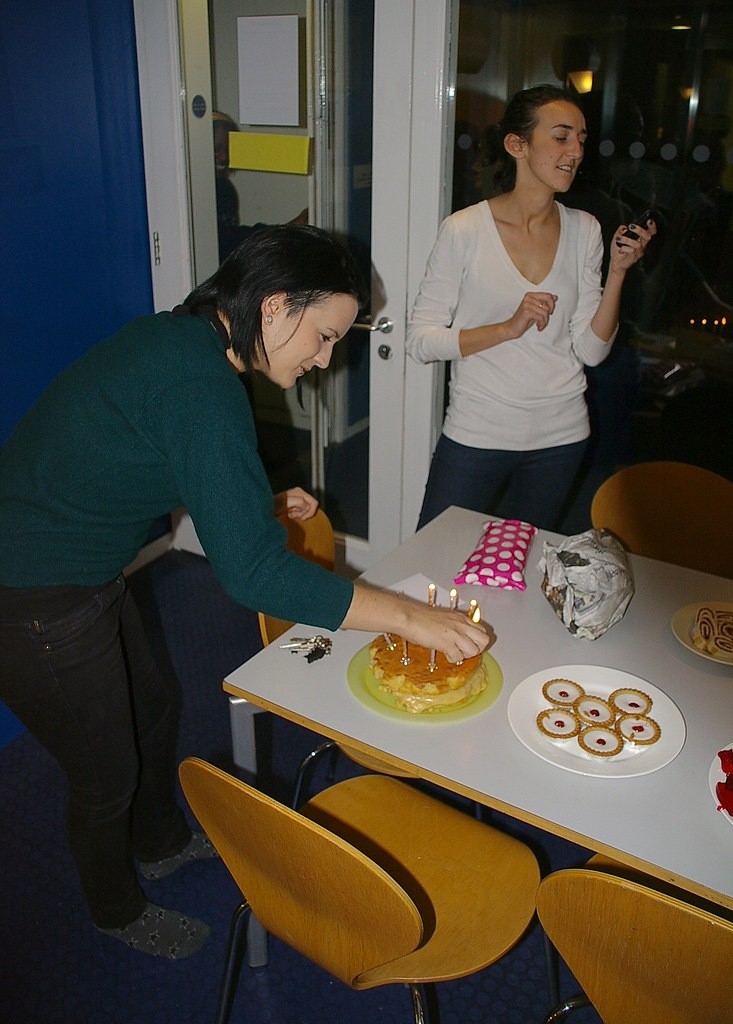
[347,641,503,724]
[709,742,733,826]
[670,601,733,665]
[507,665,687,778]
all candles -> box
[380,584,480,670]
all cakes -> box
[368,632,486,712]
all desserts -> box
[536,679,662,761]
[690,607,733,660]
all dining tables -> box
[222,504,733,968]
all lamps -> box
[563,38,593,95]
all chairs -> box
[258,503,482,816]
[593,459,733,580]
[179,739,563,1023]
[535,853,733,1024]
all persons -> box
[0,223,491,959]
[212,110,242,265]
[403,85,656,533]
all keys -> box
[279,634,333,664]
